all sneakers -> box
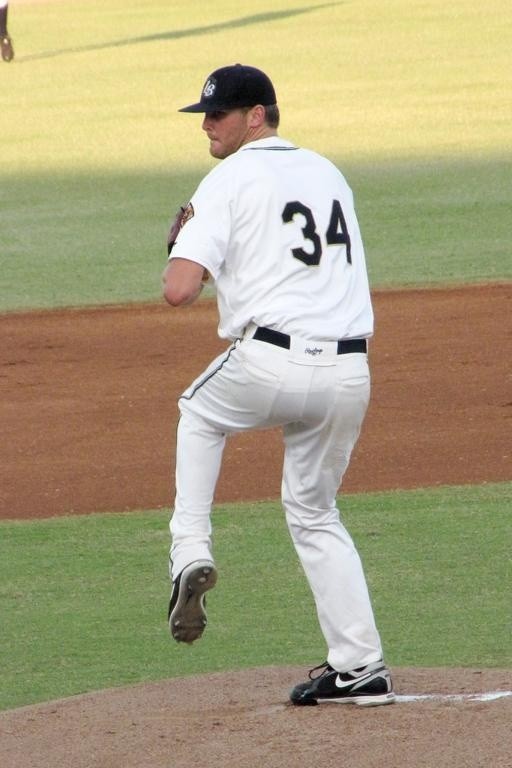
[290,659,395,707]
[168,559,217,643]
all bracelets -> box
[168,241,175,256]
[0,36,11,46]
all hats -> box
[179,63,276,112]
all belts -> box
[254,328,366,354]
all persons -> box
[0,1,17,62]
[162,63,396,708]
